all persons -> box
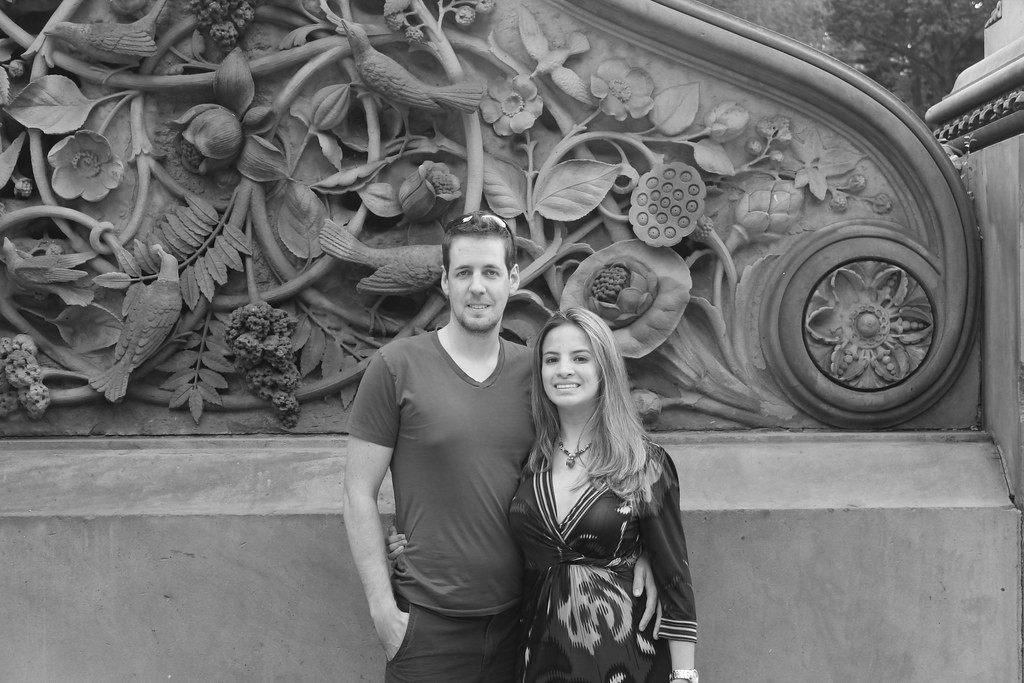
[388,306,699,683]
[345,210,532,683]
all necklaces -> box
[557,433,592,469]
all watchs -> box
[669,668,698,683]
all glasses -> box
[443,214,516,250]
